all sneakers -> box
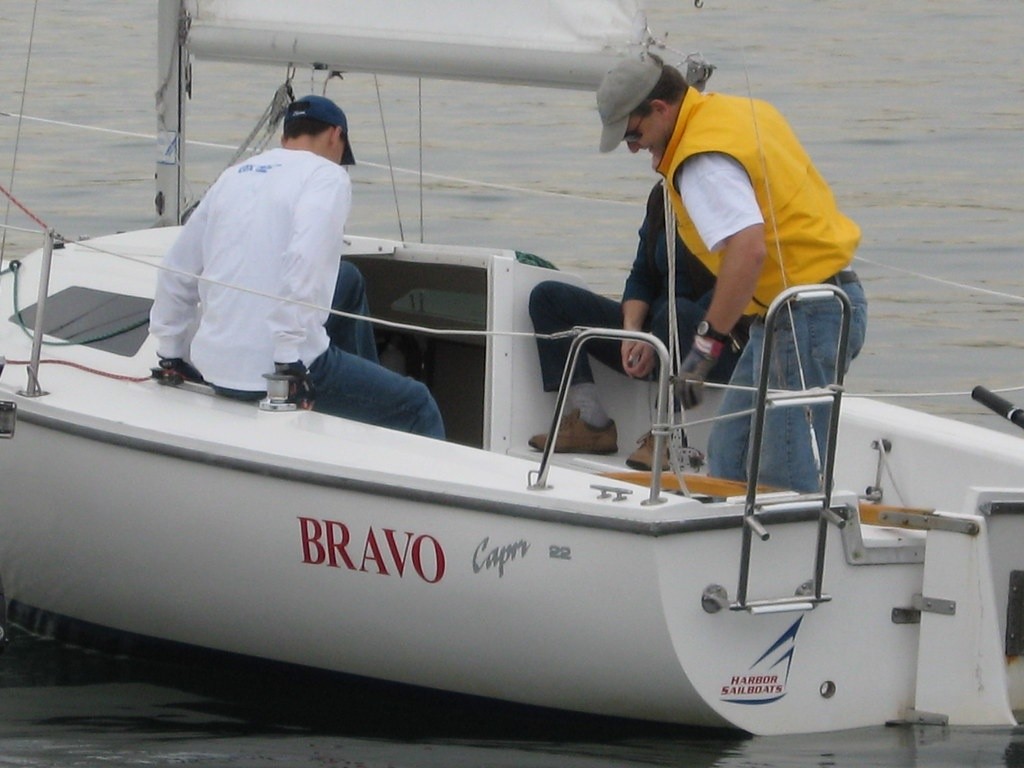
[528,409,618,454]
[626,430,688,470]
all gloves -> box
[655,334,726,412]
[158,353,209,386]
[276,359,315,411]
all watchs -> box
[695,320,730,344]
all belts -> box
[823,271,859,286]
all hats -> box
[596,51,662,152]
[284,95,356,166]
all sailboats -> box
[0,0,1024,737]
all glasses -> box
[622,116,644,142]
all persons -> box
[147,96,444,441]
[597,57,867,495]
[529,154,750,471]
